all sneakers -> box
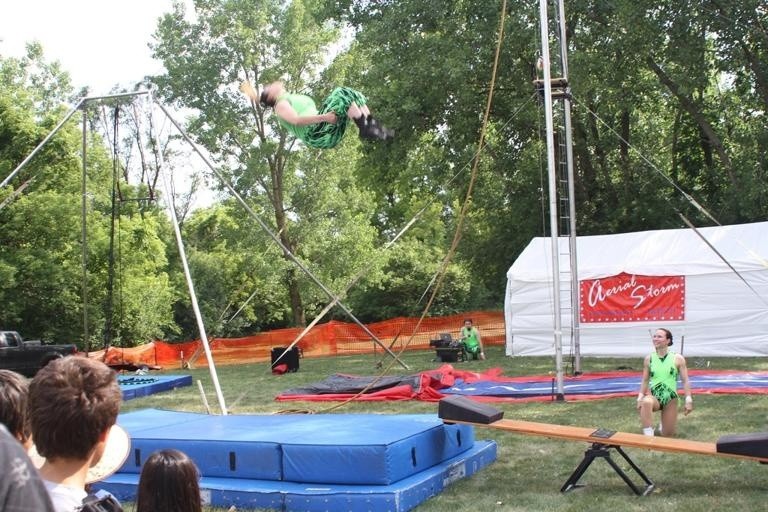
[369,117,395,138]
[358,120,386,141]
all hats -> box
[26,422,131,485]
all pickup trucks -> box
[0,331,77,379]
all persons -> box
[636,329,693,436]
[0,369,32,452]
[0,421,56,511]
[259,79,394,150]
[28,355,121,510]
[458,318,485,361]
[135,448,202,512]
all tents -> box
[501,219,768,360]
